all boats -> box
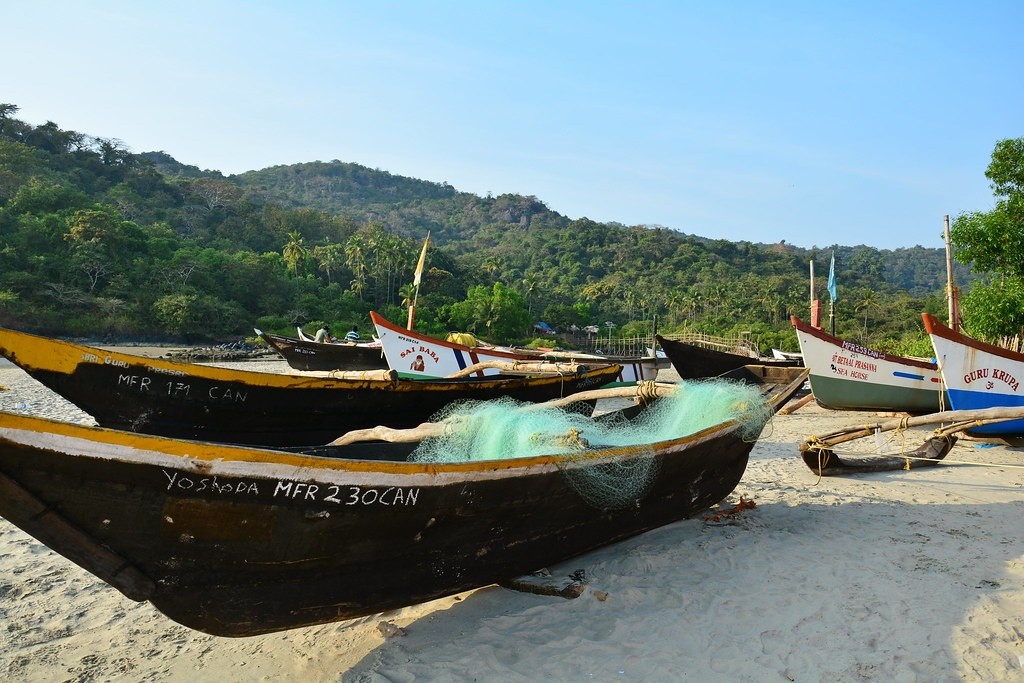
[370,312,659,383]
[790,251,951,411]
[921,215,1024,437]
[0,362,814,636]
[0,328,625,462]
[656,334,804,386]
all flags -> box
[827,254,837,301]
[414,239,428,285]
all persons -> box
[344,325,360,340]
[314,325,330,343]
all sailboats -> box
[256,230,432,371]
